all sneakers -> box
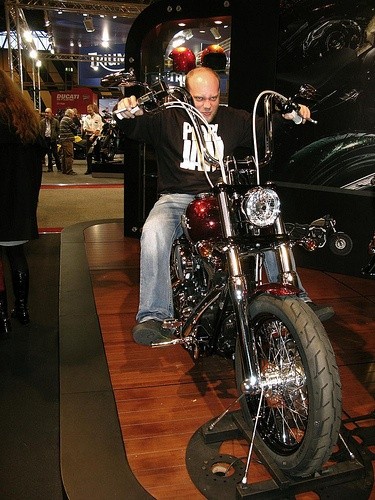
[305,301,335,322]
[132,319,173,347]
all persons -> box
[112,68,336,348]
[40,103,106,176]
[0,68,47,340]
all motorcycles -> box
[101,68,342,480]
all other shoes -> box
[47,169,53,172]
[84,170,92,175]
[69,172,78,175]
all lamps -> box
[84,14,95,33]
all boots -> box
[12,264,29,325]
[0,289,12,337]
